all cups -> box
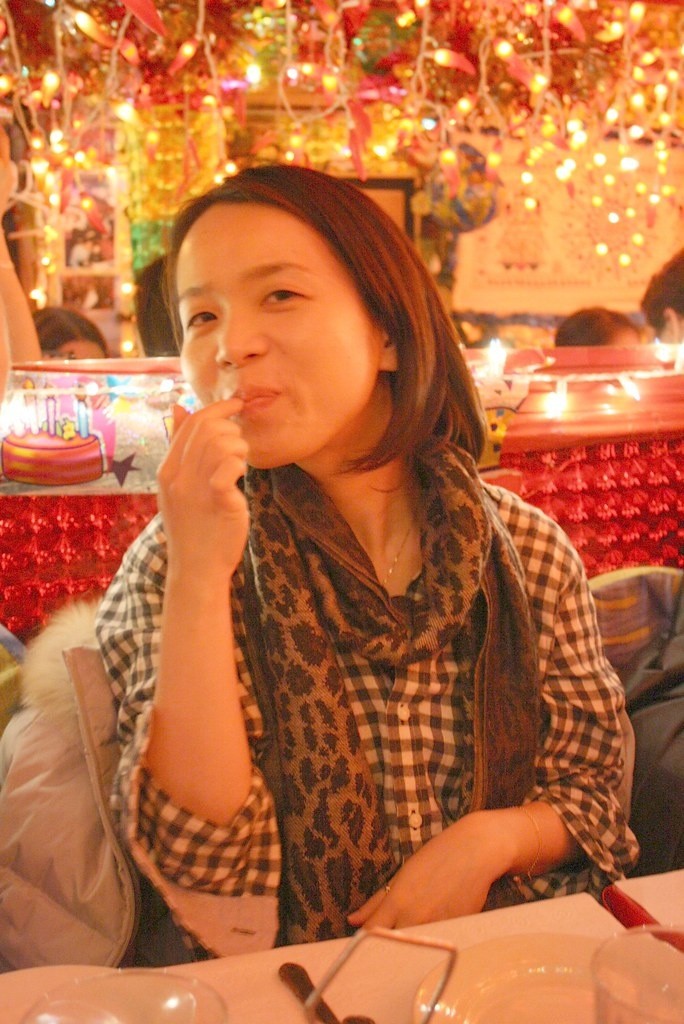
[21,972,231,1024]
[592,918,684,1024]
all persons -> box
[557,307,642,347]
[131,254,181,357]
[642,248,684,345]
[97,166,640,964]
[34,306,108,359]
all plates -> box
[2,965,131,1021]
[412,932,608,1023]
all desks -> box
[0,869,684,1024]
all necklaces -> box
[381,515,417,591]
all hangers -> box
[0,155,114,241]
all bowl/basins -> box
[617,369,684,406]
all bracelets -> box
[511,807,542,878]
